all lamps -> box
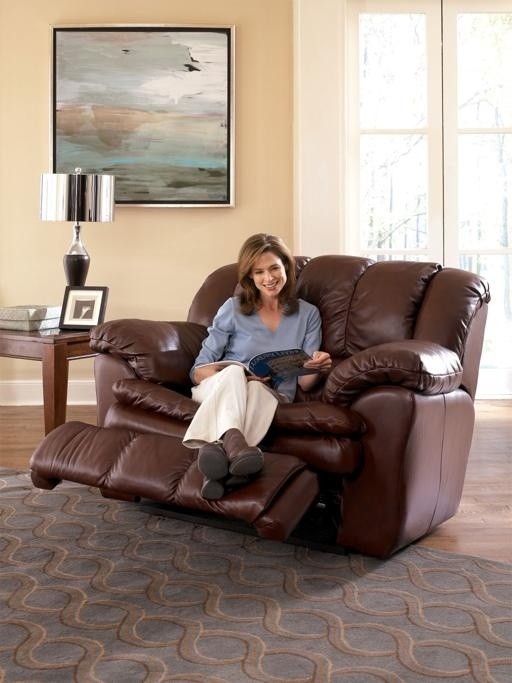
[39,172,116,285]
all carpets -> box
[2,417,512,680]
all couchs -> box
[29,253,490,559]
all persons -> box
[182,232,332,499]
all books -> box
[197,348,318,389]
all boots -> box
[222,428,264,489]
[198,443,229,502]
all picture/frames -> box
[49,25,237,207]
[60,285,109,330]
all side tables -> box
[0,317,98,437]
